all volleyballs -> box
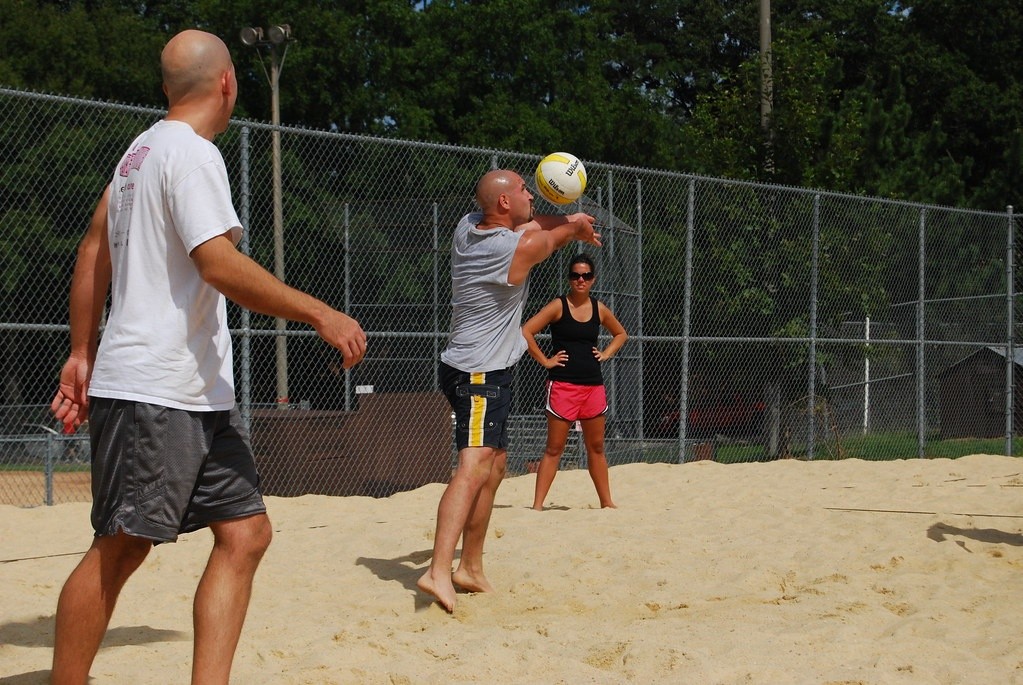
[534,151,588,205]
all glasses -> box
[570,272,594,280]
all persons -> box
[50,30,369,684]
[415,169,602,613]
[61,421,88,464]
[523,254,628,512]
